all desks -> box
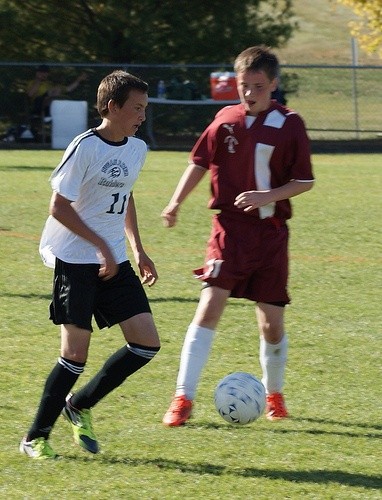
[143,96,241,148]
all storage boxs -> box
[211,73,238,99]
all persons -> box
[19,70,161,460]
[161,47,315,425]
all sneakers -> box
[19,436,63,460]
[163,396,192,427]
[62,394,99,454]
[266,393,287,422]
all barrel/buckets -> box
[51,100,88,149]
[210,72,240,100]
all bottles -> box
[158,80,165,99]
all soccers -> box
[214,371,267,425]
